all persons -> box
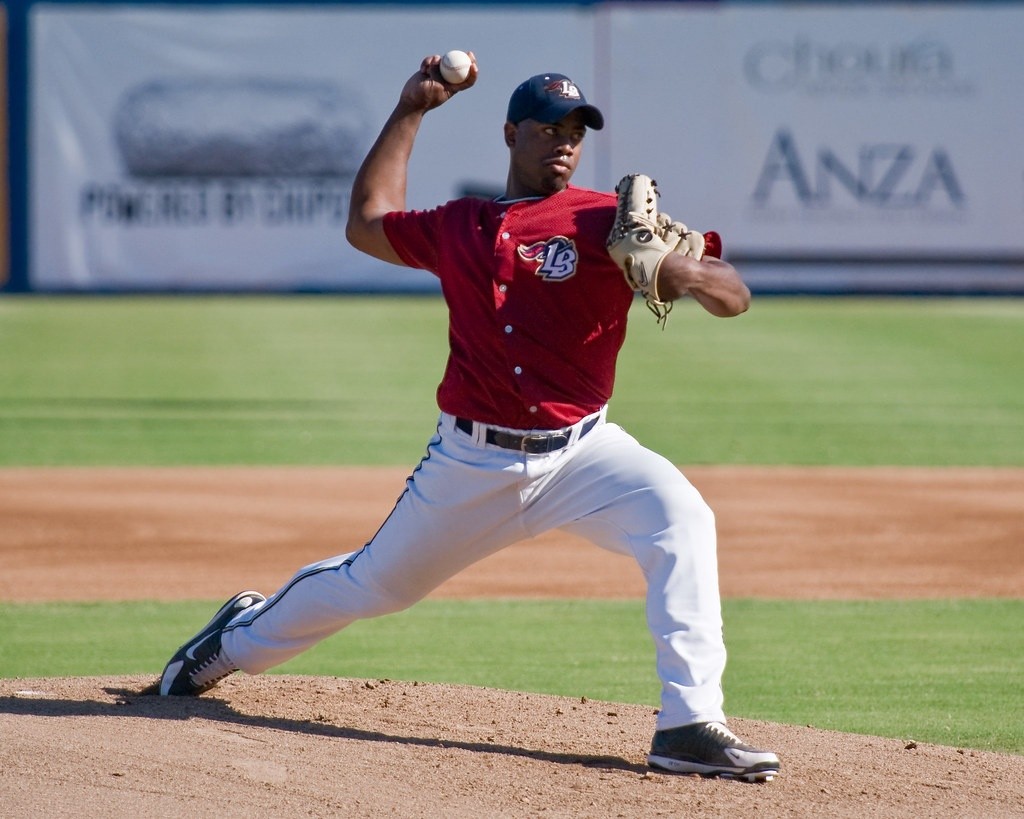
[160,49,780,785]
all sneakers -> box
[158,591,268,699]
[647,719,780,783]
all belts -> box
[456,416,599,456]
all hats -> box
[507,73,604,131]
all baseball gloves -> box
[604,172,724,332]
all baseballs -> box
[439,50,472,85]
